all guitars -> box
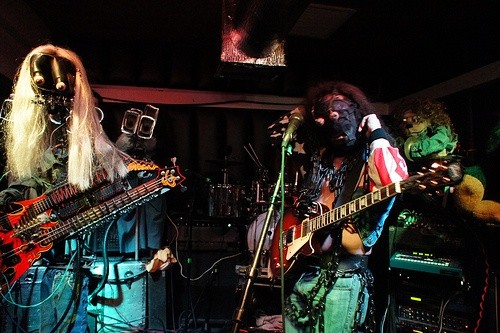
[0,163,189,294]
[245,160,466,283]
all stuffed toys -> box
[393,98,500,222]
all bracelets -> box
[368,128,386,146]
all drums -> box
[208,182,247,219]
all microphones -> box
[281,113,304,148]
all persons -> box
[0,44,130,255]
[114,134,165,253]
[285,81,409,333]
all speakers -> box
[82,250,167,333]
